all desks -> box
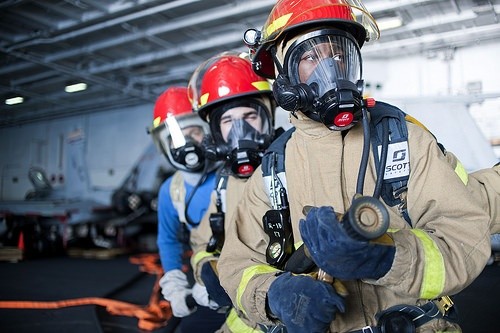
[0,201,124,249]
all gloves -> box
[299,206,396,281]
[201,262,233,307]
[267,271,349,333]
[192,283,219,310]
[158,269,197,318]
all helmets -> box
[192,55,274,123]
[152,86,197,132]
[251,0,366,79]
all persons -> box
[147,51,500,333]
[215,0,493,333]
